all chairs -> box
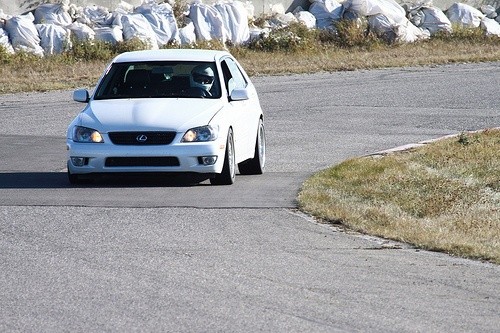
[122,69,156,96]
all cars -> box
[66,49,266,184]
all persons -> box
[189,65,214,98]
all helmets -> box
[189,65,215,91]
